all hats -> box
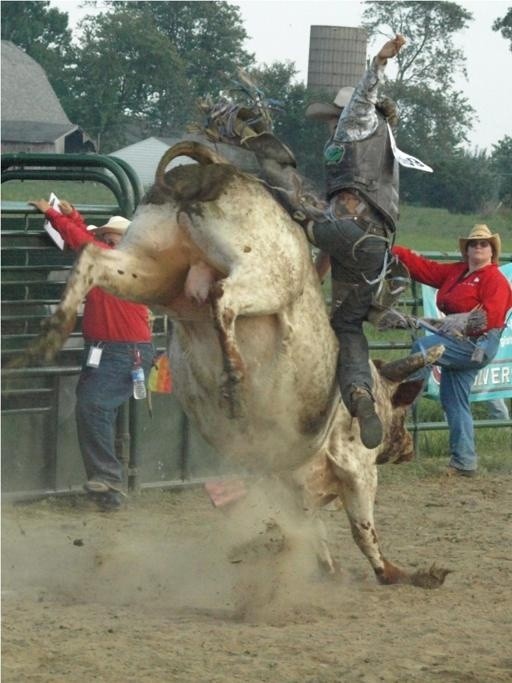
[304,86,357,117]
[459,224,501,259]
[91,216,132,235]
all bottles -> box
[129,362,147,400]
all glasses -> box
[102,239,115,248]
[470,240,489,247]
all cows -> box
[24,142,452,587]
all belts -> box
[354,215,386,237]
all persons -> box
[300,34,406,450]
[389,224,512,477]
[28,197,158,512]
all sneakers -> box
[445,465,476,477]
[352,389,382,448]
